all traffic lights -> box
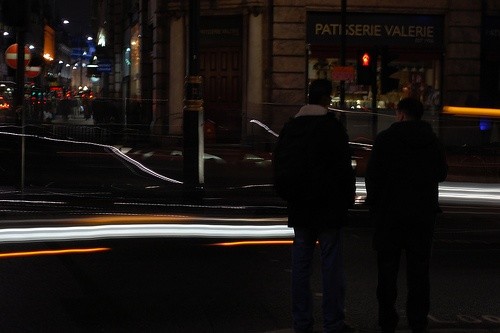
[356,50,372,84]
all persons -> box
[365,96,447,333]
[270,79,360,332]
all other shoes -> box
[328,321,354,332]
